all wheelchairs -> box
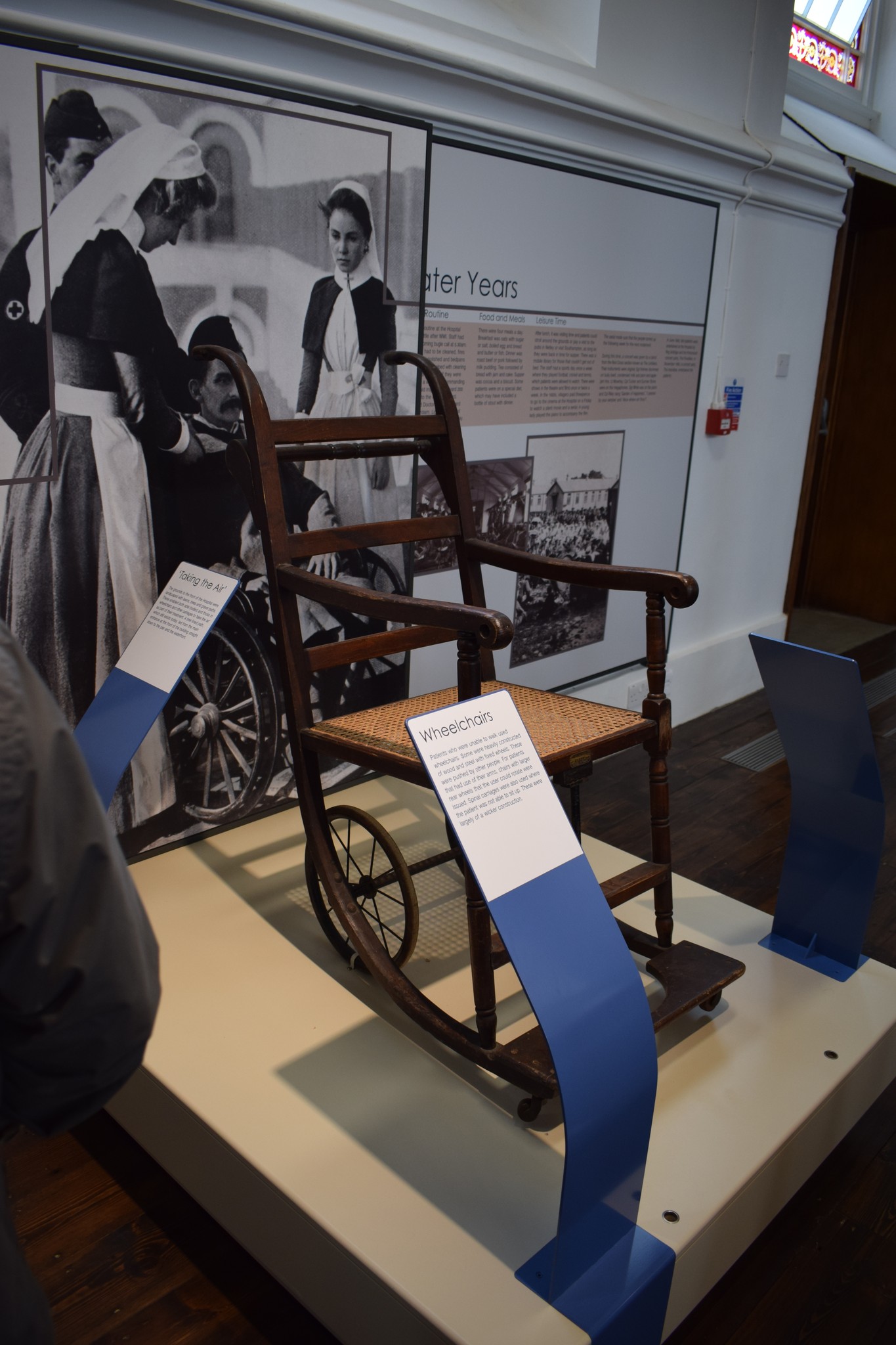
[189,341,746,1123]
[159,540,411,827]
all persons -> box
[296,182,407,687]
[0,88,345,865]
[0,620,161,1345]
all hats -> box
[26,123,206,325]
[45,88,111,141]
[329,180,387,281]
